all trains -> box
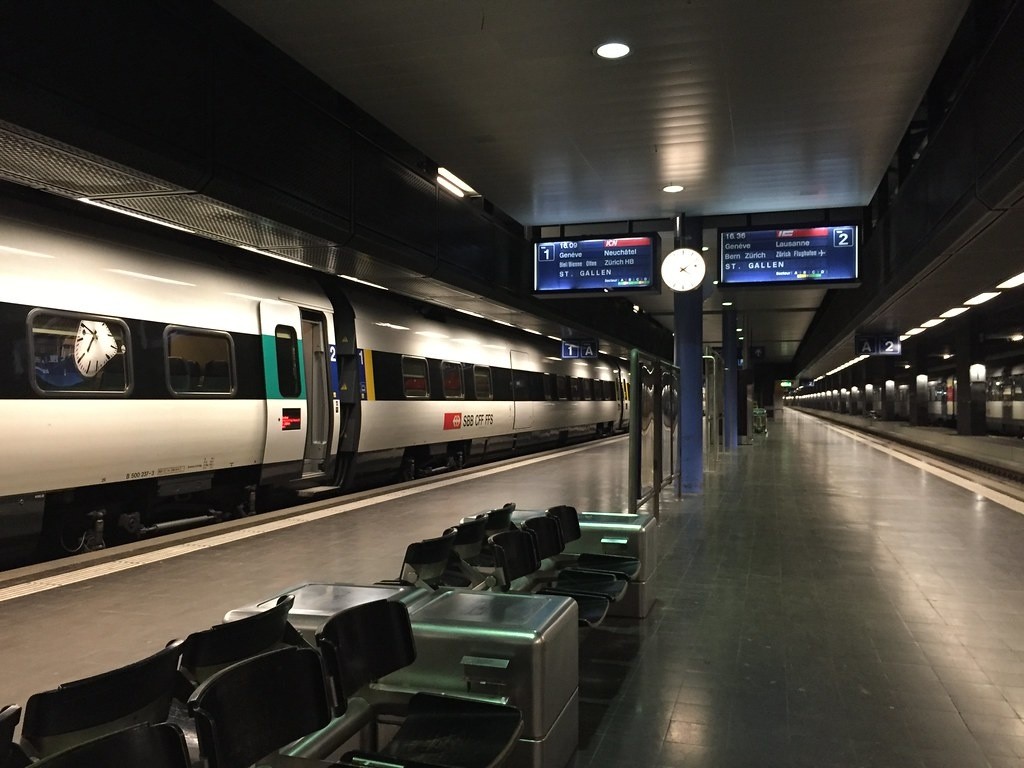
[838,364,1023,437]
[1,210,633,553]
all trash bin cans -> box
[751,408,768,438]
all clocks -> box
[660,248,707,295]
[73,319,119,381]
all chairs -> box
[0,502,644,768]
[34,357,229,392]
